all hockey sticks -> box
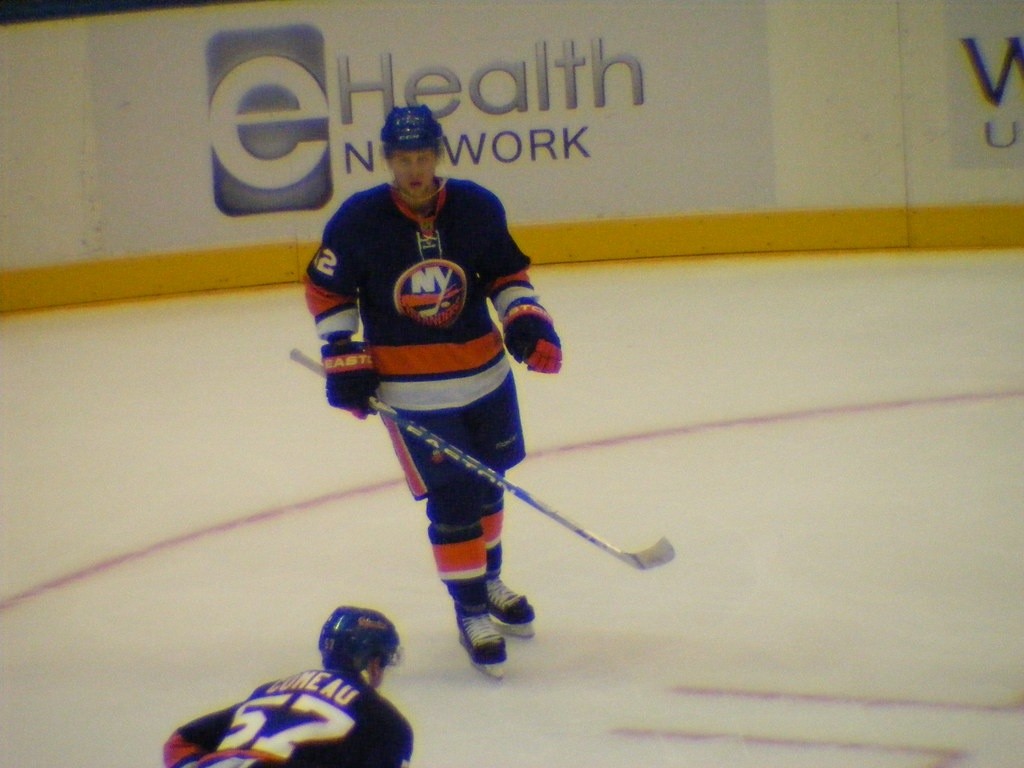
[287,345,676,575]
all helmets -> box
[320,606,399,676]
[379,104,443,160]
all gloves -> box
[321,341,380,420]
[501,302,562,374]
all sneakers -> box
[484,575,535,637]
[453,601,507,678]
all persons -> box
[303,105,562,662]
[163,607,415,768]
